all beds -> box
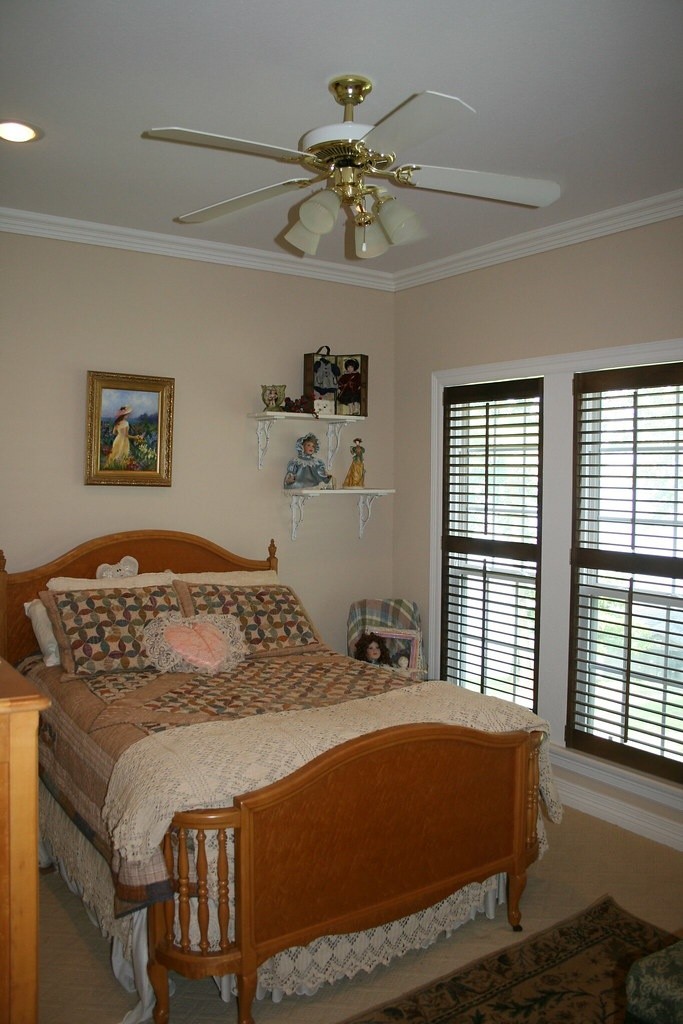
[0,530,564,1024]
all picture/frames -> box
[364,626,419,668]
[84,371,175,487]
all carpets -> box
[339,895,683,1024]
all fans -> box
[147,75,561,222]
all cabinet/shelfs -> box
[0,658,53,1024]
[247,411,395,541]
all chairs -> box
[347,599,427,681]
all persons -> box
[342,437,366,488]
[354,632,393,668]
[268,389,277,406]
[283,432,332,489]
[338,358,360,415]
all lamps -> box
[285,187,418,257]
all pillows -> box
[173,580,331,661]
[37,584,182,683]
[47,573,172,593]
[164,569,282,586]
[24,599,62,667]
[144,612,245,676]
